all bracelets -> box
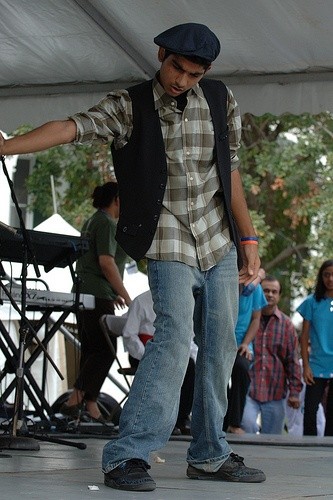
[240,240,258,248]
[257,274,263,281]
[240,237,259,241]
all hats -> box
[154,23,220,64]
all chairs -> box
[100,314,139,422]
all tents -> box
[0,213,150,419]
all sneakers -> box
[186,452,266,483]
[103,458,156,491]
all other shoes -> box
[173,425,182,434]
[60,401,107,423]
[226,426,247,434]
[174,425,191,434]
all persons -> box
[239,259,333,437]
[0,22,269,491]
